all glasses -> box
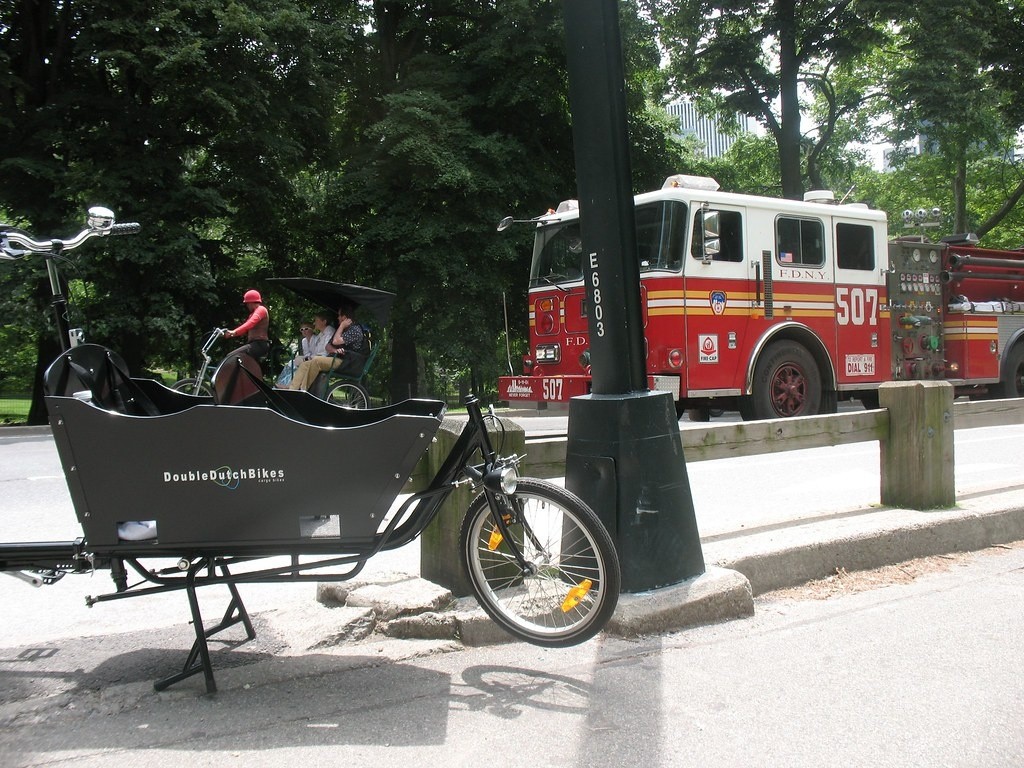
[299,326,313,332]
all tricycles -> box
[163,277,396,413]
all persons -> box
[220,289,268,358]
[274,305,364,393]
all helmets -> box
[243,289,263,304]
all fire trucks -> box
[497,172,1024,418]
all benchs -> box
[320,338,381,382]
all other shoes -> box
[274,383,289,390]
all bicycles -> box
[0,207,621,694]
